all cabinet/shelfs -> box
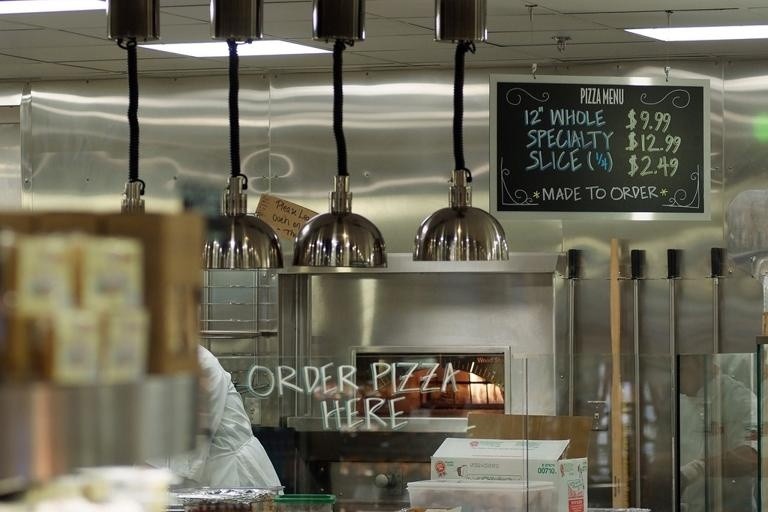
[202,269,294,432]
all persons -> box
[677,353,759,512]
[166,346,286,494]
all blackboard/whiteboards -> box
[490,72,712,223]
